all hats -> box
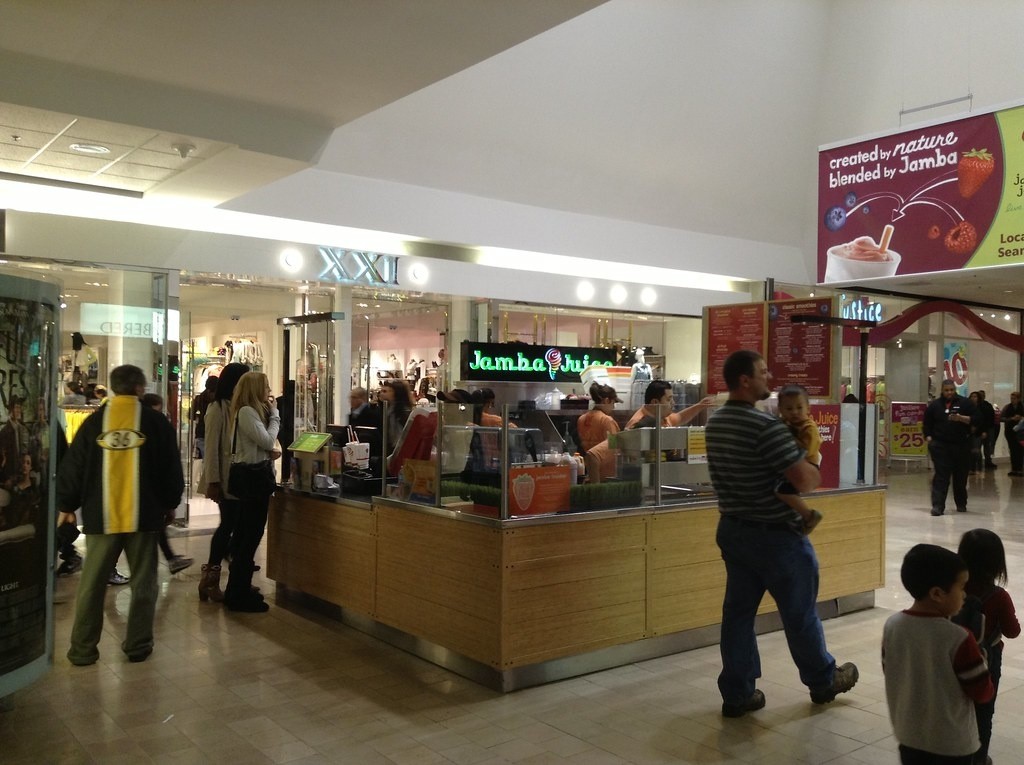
[436,389,474,405]
[600,388,624,404]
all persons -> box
[0,394,48,524]
[57,418,80,577]
[772,385,822,535]
[57,365,184,665]
[109,393,195,584]
[577,380,711,483]
[93,385,108,405]
[882,529,1021,765]
[276,380,293,483]
[704,349,860,719]
[223,373,281,613]
[921,380,1024,515]
[437,388,519,472]
[348,387,382,457]
[64,382,86,404]
[382,379,414,455]
[406,359,417,380]
[628,349,652,410]
[189,376,219,461]
[197,363,261,604]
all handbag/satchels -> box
[228,461,277,503]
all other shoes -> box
[985,461,997,468]
[1008,470,1024,476]
[932,505,944,516]
[968,470,976,474]
[224,590,269,612]
[130,648,153,662]
[957,503,967,512]
[801,510,823,536]
[57,556,83,577]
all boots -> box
[251,583,260,590]
[198,564,225,602]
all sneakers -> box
[722,689,765,718]
[108,569,130,584]
[170,555,193,574]
[810,662,859,704]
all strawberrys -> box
[957,147,995,198]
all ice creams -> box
[830,224,896,262]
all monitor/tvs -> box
[287,431,332,455]
[327,424,383,456]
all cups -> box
[342,441,370,469]
[824,245,901,283]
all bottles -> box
[559,452,585,486]
[552,387,560,410]
